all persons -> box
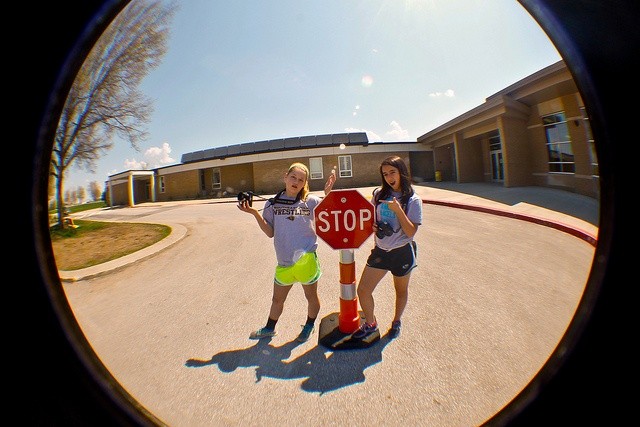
[350,155,423,339]
[236,161,337,343]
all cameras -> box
[376,221,393,240]
[236,191,253,208]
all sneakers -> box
[297,324,316,342]
[389,320,401,339]
[250,328,276,338]
[352,316,378,341]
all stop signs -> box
[313,189,376,251]
